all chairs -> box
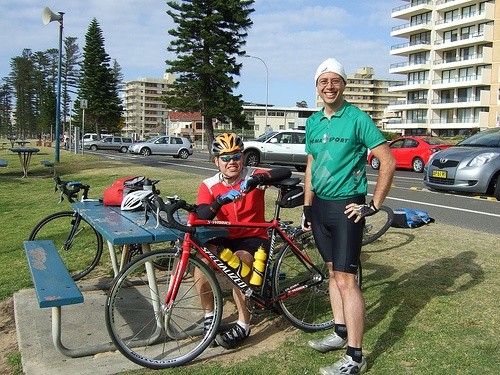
[283,138,288,142]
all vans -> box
[79,133,113,146]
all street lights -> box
[243,54,269,133]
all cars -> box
[367,135,456,173]
[423,127,500,202]
[128,135,193,159]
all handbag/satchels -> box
[103,176,133,205]
[122,176,146,198]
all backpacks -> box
[389,208,430,228]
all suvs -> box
[241,129,308,173]
[83,136,133,153]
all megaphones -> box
[41,6,59,26]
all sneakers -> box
[308,331,347,352]
[204,317,220,347]
[215,323,251,350]
[319,354,368,375]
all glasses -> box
[317,78,343,87]
[218,153,243,162]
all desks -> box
[8,147,40,178]
[72,202,190,346]
[15,141,30,147]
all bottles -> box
[248,243,267,286]
[215,245,251,278]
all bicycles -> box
[269,204,395,253]
[29,175,198,283]
[104,176,364,371]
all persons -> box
[194,132,293,350]
[301,58,396,375]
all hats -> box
[315,58,347,88]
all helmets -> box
[211,132,244,157]
[120,190,156,210]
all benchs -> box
[0,160,8,167]
[41,161,54,177]
[23,240,83,358]
